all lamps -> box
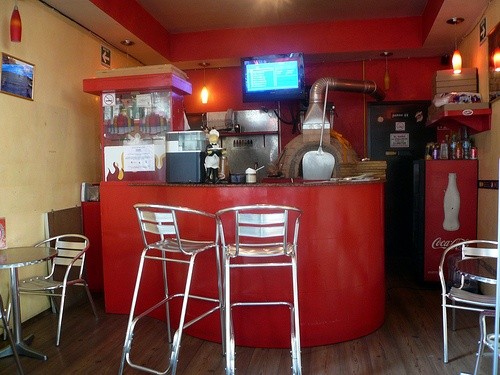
[447,17,464,73]
[10,0,22,43]
[381,52,392,90]
[200,62,210,103]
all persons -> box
[204,129,223,185]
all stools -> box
[120,204,224,375]
[215,205,302,375]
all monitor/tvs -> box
[241,52,305,103]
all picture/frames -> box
[0,52,35,101]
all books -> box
[336,161,387,180]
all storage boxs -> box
[430,69,478,96]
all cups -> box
[245,167,257,184]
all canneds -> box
[470,147,476,159]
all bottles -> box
[430,140,471,160]
[107,105,167,135]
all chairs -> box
[439,239,498,363]
[7,235,98,347]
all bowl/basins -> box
[229,173,247,184]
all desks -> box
[0,247,57,361]
